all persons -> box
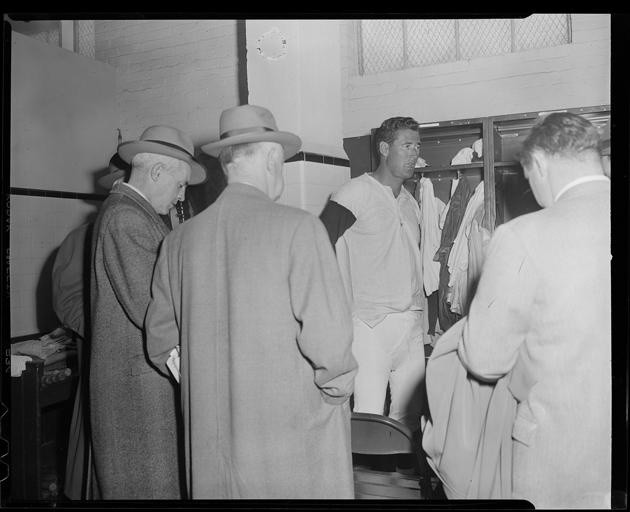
[460,114,611,511]
[142,103,357,498]
[89,125,207,500]
[50,153,131,500]
[318,117,427,467]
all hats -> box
[202,105,302,160]
[98,125,207,188]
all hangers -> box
[396,168,466,191]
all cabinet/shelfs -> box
[369,103,488,356]
[5,329,80,508]
[491,105,608,228]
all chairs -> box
[346,408,446,506]
